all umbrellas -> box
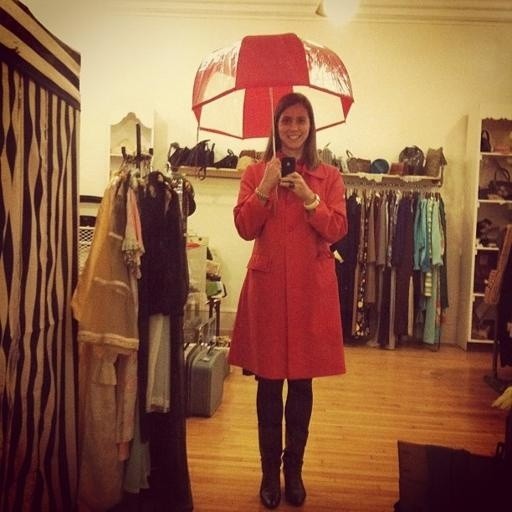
[191,32,356,160]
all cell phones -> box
[280,156,295,177]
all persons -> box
[227,95,350,509]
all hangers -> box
[345,179,442,206]
[112,154,179,198]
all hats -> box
[183,179,196,216]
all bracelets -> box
[303,194,321,212]
[255,188,270,201]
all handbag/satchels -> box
[318,145,446,177]
[481,131,491,152]
[489,168,512,200]
[169,140,266,170]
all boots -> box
[282,430,308,506]
[260,429,281,509]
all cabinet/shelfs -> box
[107,110,170,179]
[463,112,512,352]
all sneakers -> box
[477,218,500,247]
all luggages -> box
[184,316,231,417]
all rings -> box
[288,182,295,188]
